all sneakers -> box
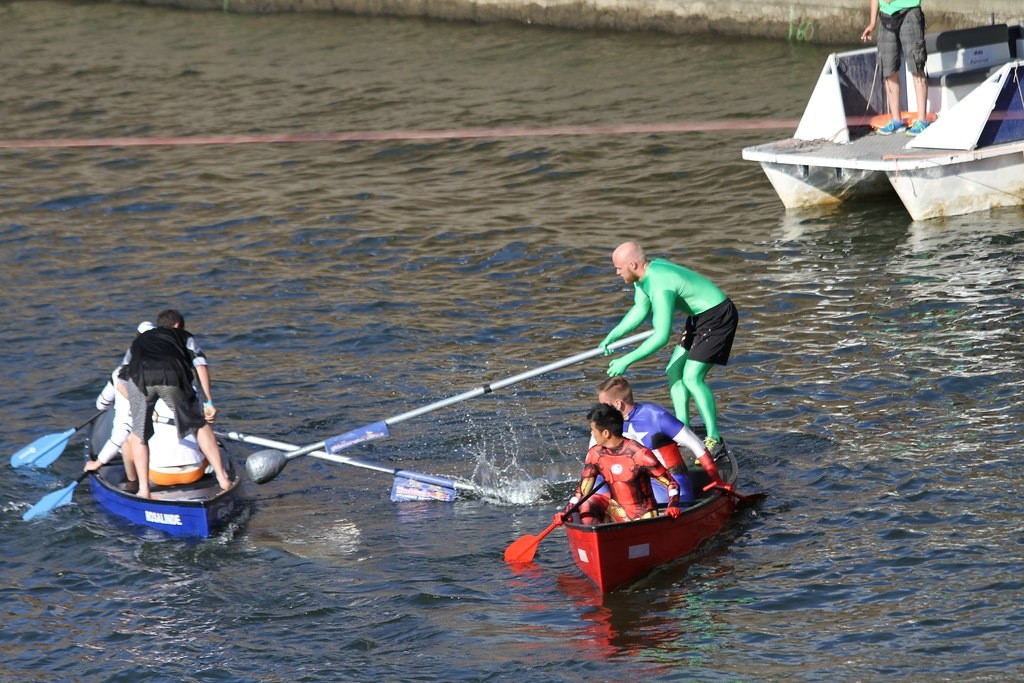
[877,120,907,134]
[905,121,930,136]
[694,436,726,464]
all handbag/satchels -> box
[878,6,911,31]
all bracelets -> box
[203,402,213,406]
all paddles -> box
[703,481,769,504]
[11,409,107,469]
[504,481,606,563]
[23,469,92,522]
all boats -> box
[83,383,246,539]
[742,24,1024,220]
[560,415,739,593]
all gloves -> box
[554,512,573,525]
[703,480,732,492]
[664,507,681,518]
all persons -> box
[128,310,233,498]
[861,0,929,136]
[589,376,733,516]
[83,321,230,486]
[553,403,681,525]
[599,242,739,465]
